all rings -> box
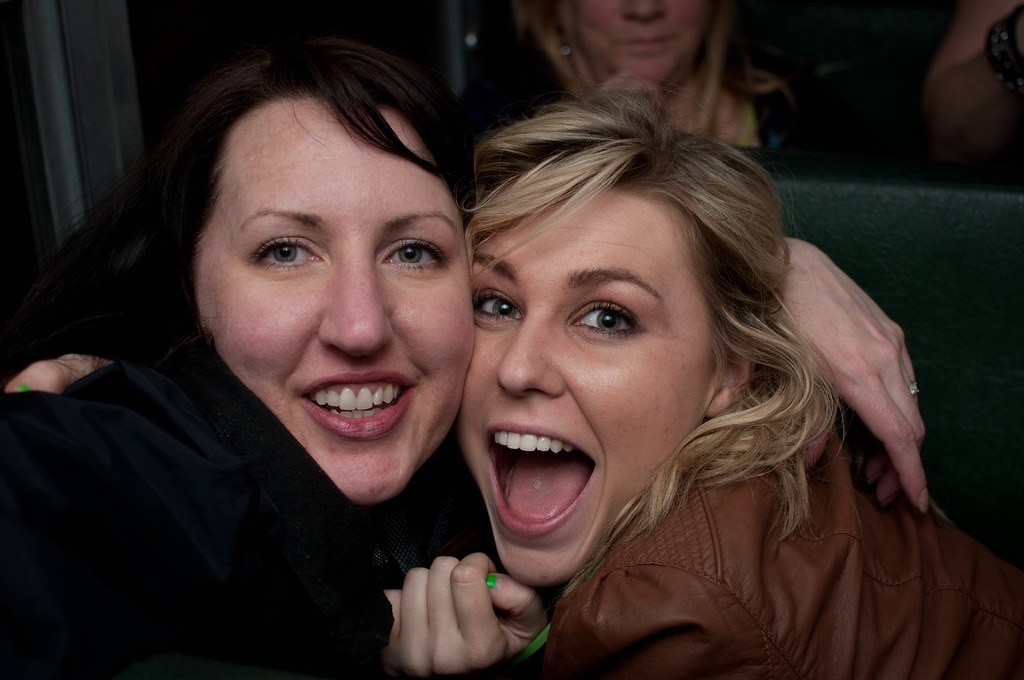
[908,382,919,395]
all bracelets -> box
[985,5,1024,91]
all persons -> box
[920,0,1024,160]
[0,0,926,680]
[368,90,1024,680]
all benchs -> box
[739,0,1024,569]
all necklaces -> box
[514,623,551,664]
